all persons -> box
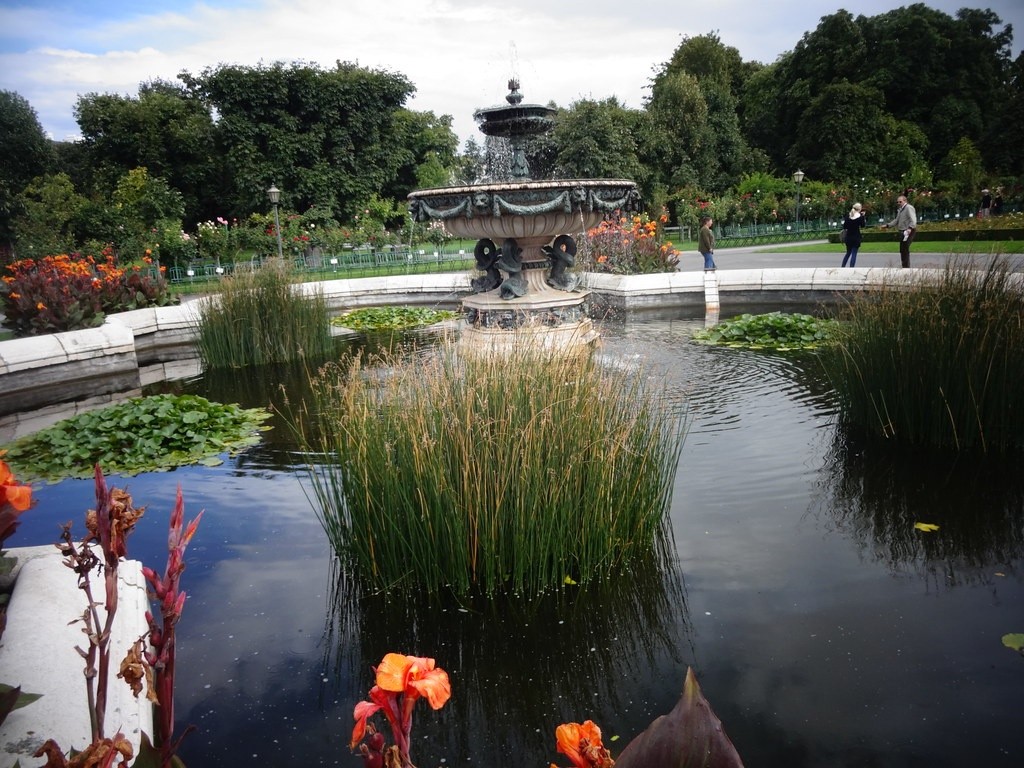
[698,217,716,271]
[993,189,1003,216]
[880,196,917,268]
[841,203,866,267]
[979,188,993,220]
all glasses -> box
[896,201,904,204]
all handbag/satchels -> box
[839,228,847,242]
